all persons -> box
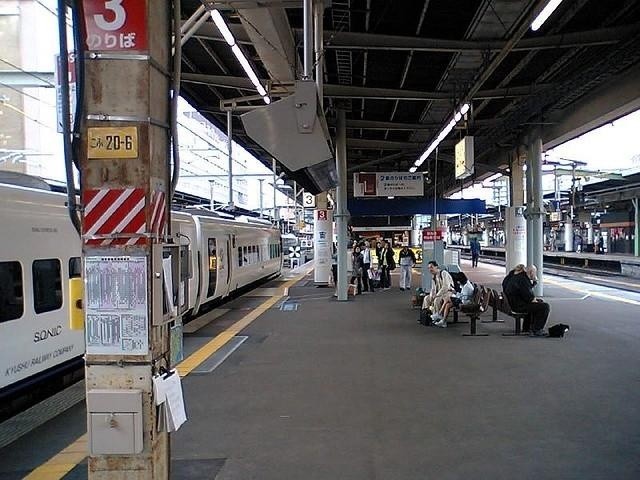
[332,237,395,296]
[501,264,530,332]
[488,225,622,253]
[430,271,474,328]
[397,242,417,291]
[417,260,455,324]
[470,237,481,266]
[503,263,550,337]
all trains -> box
[0,168,284,420]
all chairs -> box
[454,282,530,336]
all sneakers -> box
[430,311,442,319]
[356,284,410,295]
[535,329,548,336]
[529,329,536,337]
[434,319,447,328]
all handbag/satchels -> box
[461,304,479,313]
[545,324,569,338]
[417,307,432,326]
